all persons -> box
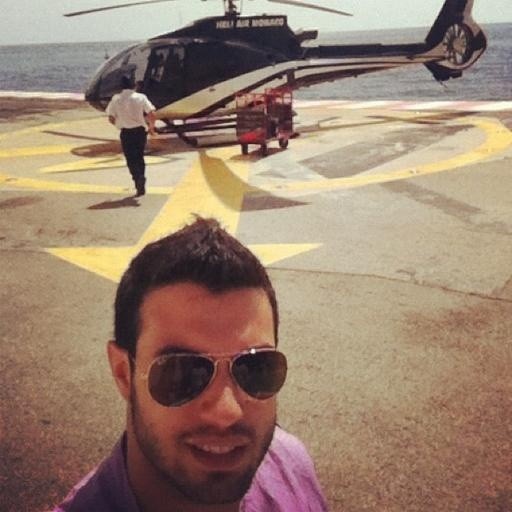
[106,74,157,197]
[50,215,328,511]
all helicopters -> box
[64,0,488,148]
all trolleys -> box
[235,87,298,156]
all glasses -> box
[131,346,288,409]
